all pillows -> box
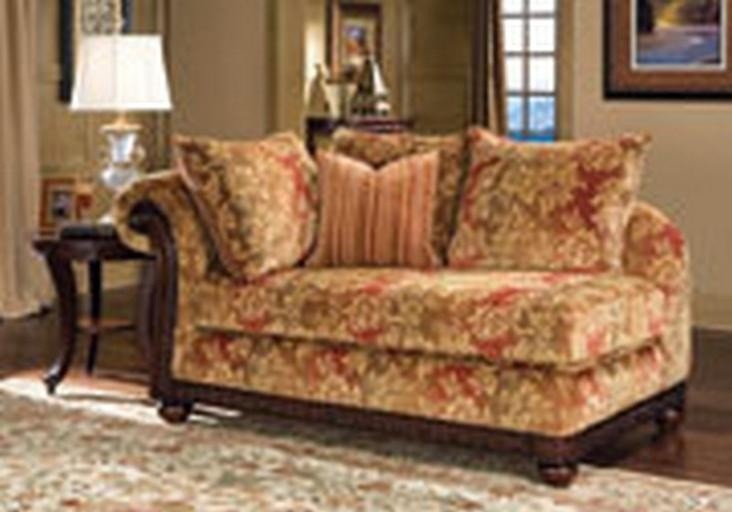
[328,127,467,268]
[446,125,652,274]
[305,144,441,267]
[167,130,320,281]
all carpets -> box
[1,364,732,512]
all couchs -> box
[111,124,695,488]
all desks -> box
[306,117,414,155]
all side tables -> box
[30,217,153,393]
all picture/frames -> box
[602,0,732,101]
[56,0,131,104]
[41,175,78,228]
[328,1,384,84]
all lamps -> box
[69,34,175,224]
